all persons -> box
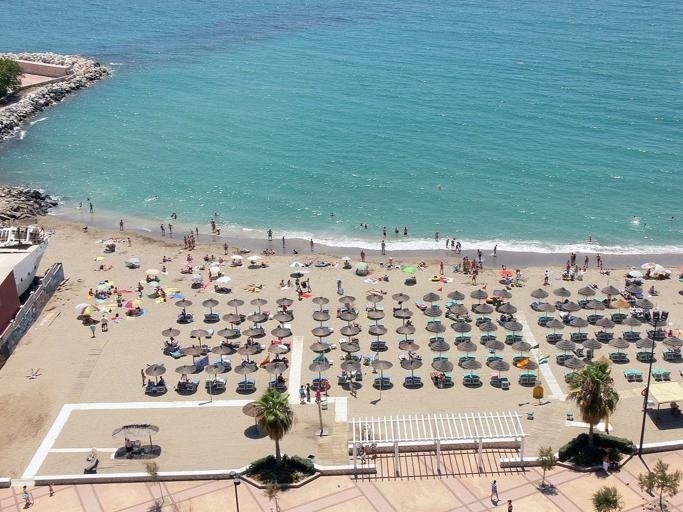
[139,369,144,387]
[124,438,133,457]
[315,390,322,405]
[299,387,307,404]
[507,500,513,512]
[502,264,521,283]
[646,269,650,277]
[490,479,502,503]
[307,384,311,400]
[280,213,498,294]
[48,480,54,496]
[23,485,32,508]
[79,196,275,336]
[544,253,603,284]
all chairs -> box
[308,299,539,397]
[143,299,295,397]
[530,299,682,398]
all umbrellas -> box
[629,270,643,280]
[641,261,655,273]
